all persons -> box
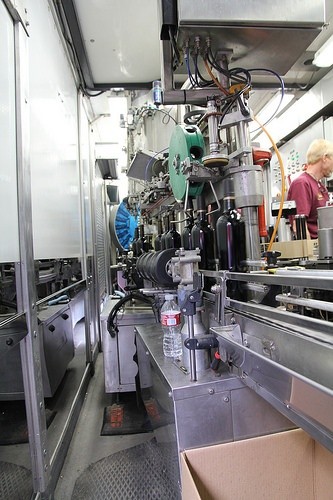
[286,138,333,262]
